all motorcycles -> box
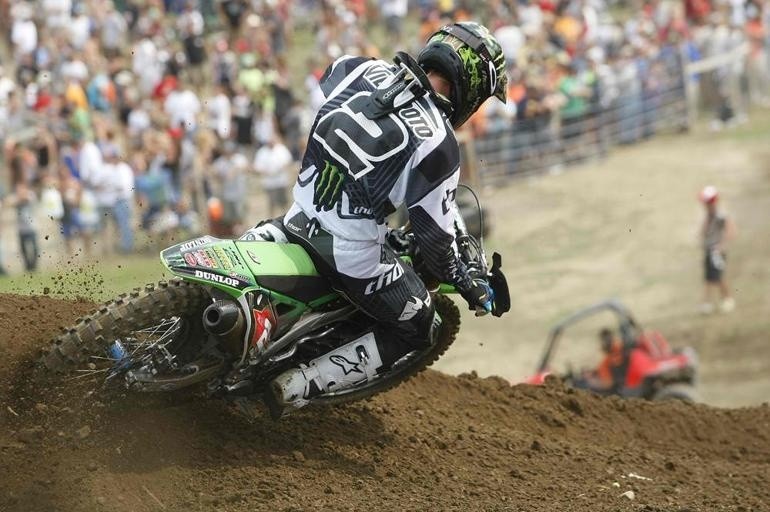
[28,180,512,422]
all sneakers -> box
[268,363,321,423]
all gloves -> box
[463,278,494,316]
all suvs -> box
[511,296,698,410]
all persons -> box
[232,20,510,420]
[0,1,769,275]
[695,186,736,315]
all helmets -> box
[417,21,507,130]
